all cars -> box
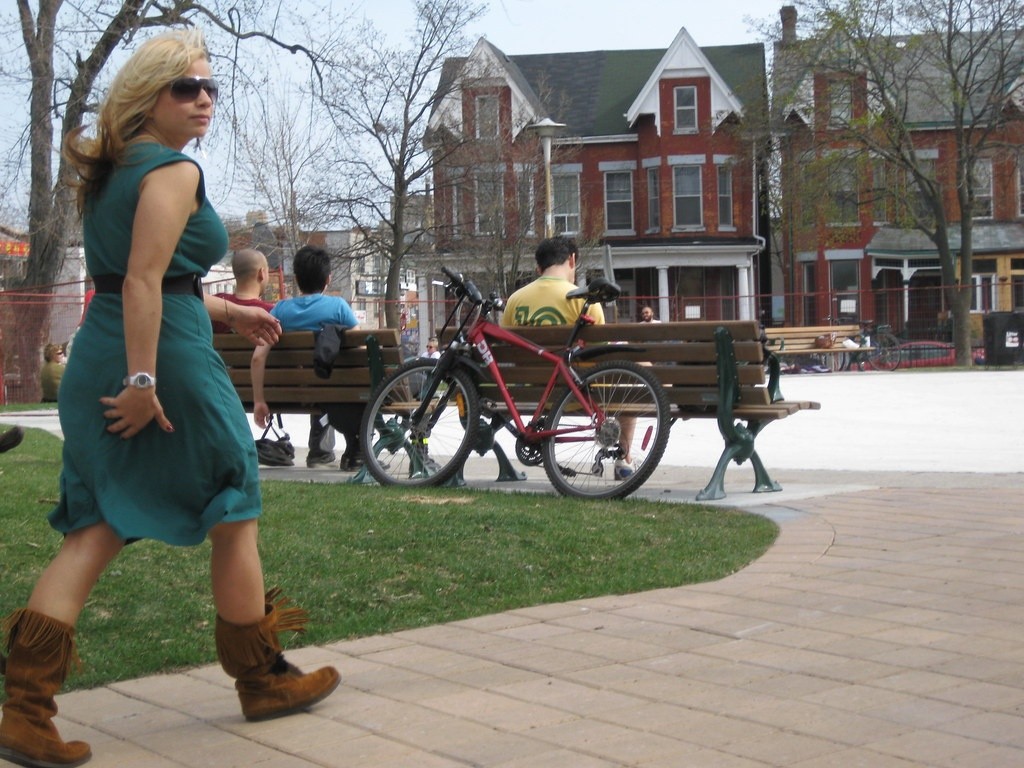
[849,339,985,372]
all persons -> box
[40,343,67,403]
[501,236,638,480]
[250,244,386,471]
[0,424,23,455]
[639,306,661,324]
[422,337,441,359]
[0,24,341,766]
[211,249,276,407]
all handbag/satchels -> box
[254,425,294,464]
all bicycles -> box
[819,313,902,373]
[356,265,673,503]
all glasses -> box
[56,352,63,355]
[167,79,219,105]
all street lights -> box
[525,117,568,239]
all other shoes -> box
[0,427,24,454]
[615,466,636,481]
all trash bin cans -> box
[984,310,1024,365]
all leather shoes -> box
[340,455,390,472]
[306,451,335,468]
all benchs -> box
[211,328,450,484]
[434,319,820,502]
[765,324,876,373]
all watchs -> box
[123,372,157,389]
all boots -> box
[0,608,91,768]
[216,589,341,722]
